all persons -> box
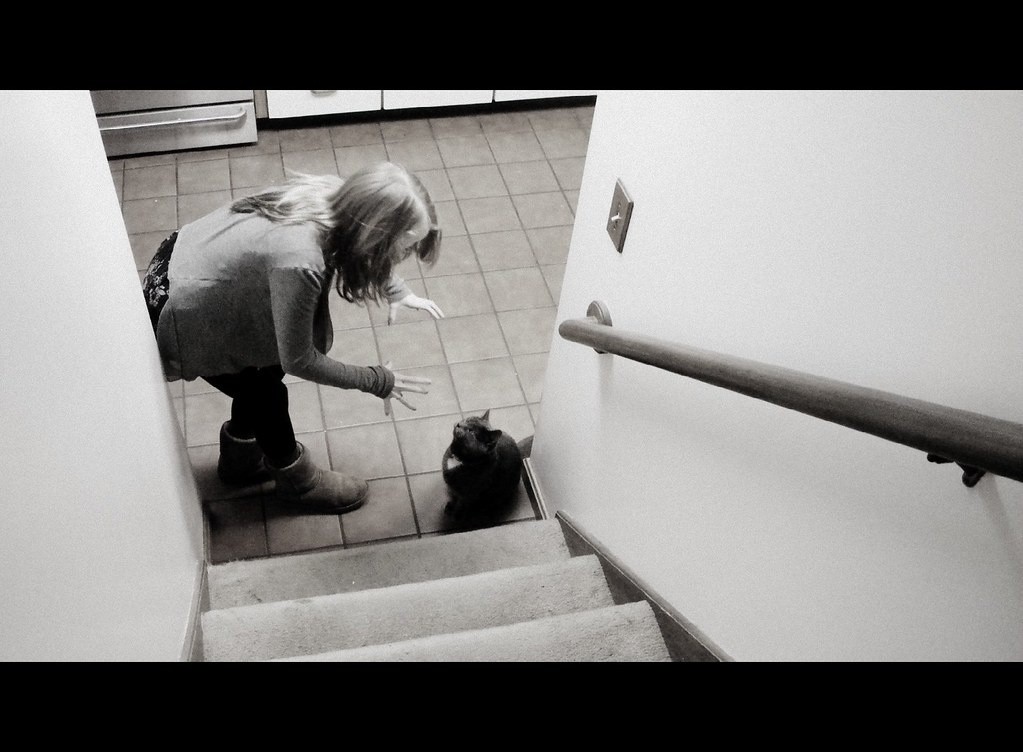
[143,161,445,515]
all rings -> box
[397,393,403,400]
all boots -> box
[263,441,369,511]
[218,420,272,485]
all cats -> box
[442,408,522,503]
